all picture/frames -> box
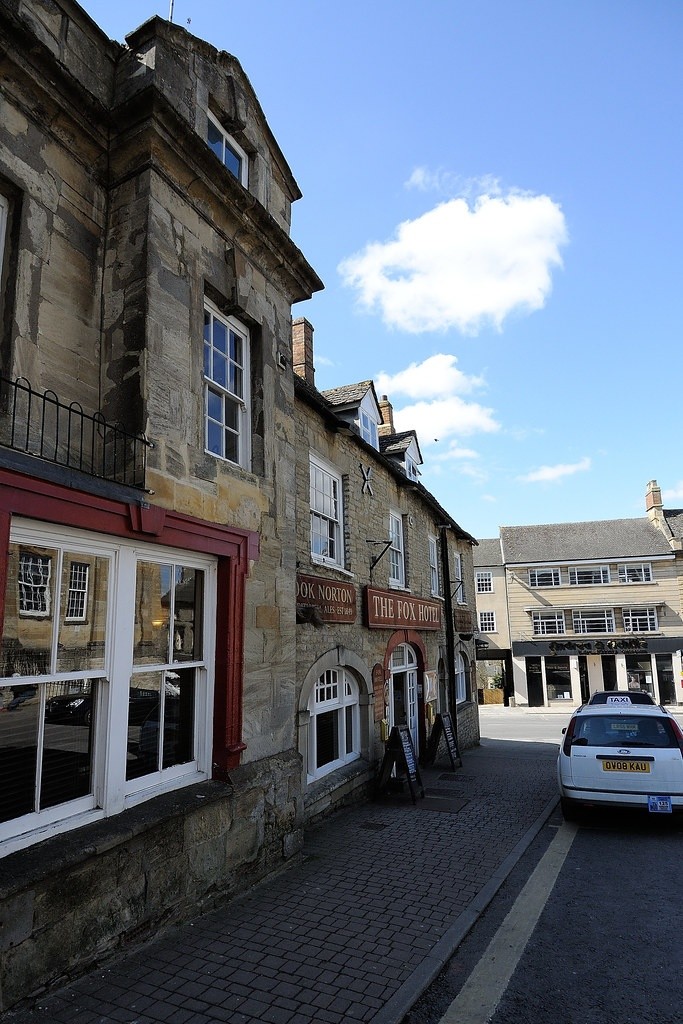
[628,674,640,689]
[424,669,438,703]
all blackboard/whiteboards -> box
[376,725,424,797]
[426,712,460,761]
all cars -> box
[555,691,683,824]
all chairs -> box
[589,718,606,734]
[638,720,659,736]
